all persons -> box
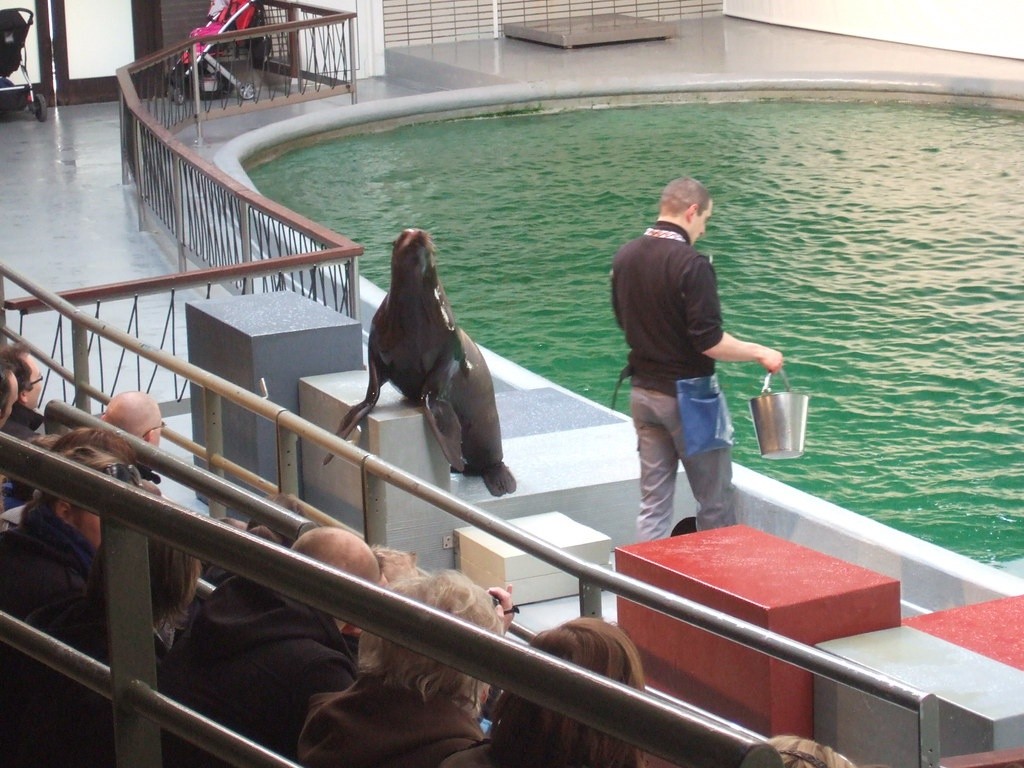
[611,177,783,542]
[1,340,419,768]
[739,736,855,768]
[296,569,513,768]
[437,618,647,768]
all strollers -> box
[165,0,273,104]
[0,7,48,123]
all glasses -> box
[103,463,144,488]
[142,421,167,438]
[29,372,43,386]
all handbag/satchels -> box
[675,373,736,458]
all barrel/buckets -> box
[750,368,809,459]
